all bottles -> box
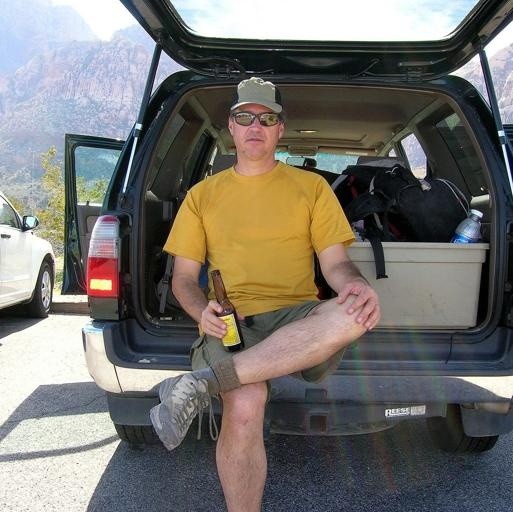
[210,269,245,352]
[450,209,484,244]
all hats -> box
[230,76,283,113]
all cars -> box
[0,192,55,318]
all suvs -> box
[60,0,513,452]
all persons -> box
[149,75,383,512]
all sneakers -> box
[148,374,213,450]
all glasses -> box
[233,112,280,126]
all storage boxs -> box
[346,241,489,328]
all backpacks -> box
[370,164,469,243]
